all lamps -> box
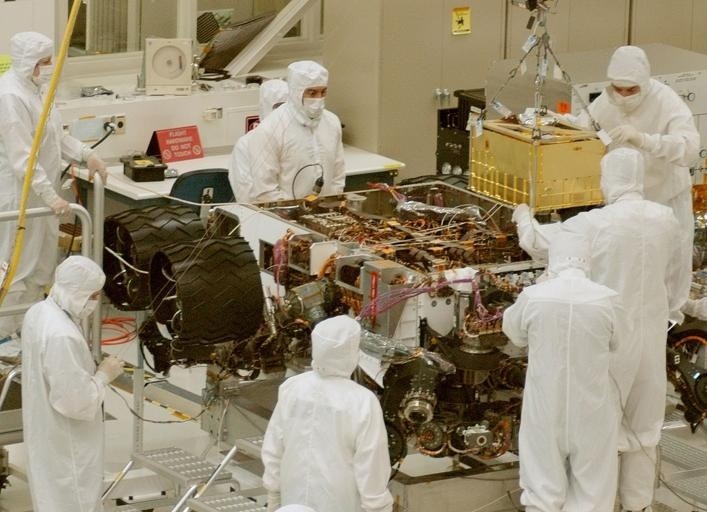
[292,163,324,200]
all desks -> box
[61,142,405,226]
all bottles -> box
[434,89,450,109]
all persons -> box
[260,314,396,512]
[0,30,109,299]
[249,60,347,200]
[227,79,285,203]
[510,148,689,512]
[20,255,124,512]
[502,230,641,512]
[562,45,700,326]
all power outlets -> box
[100,116,112,135]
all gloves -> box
[607,125,644,150]
[97,355,128,383]
[86,153,109,186]
[510,202,541,222]
[49,196,71,217]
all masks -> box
[31,64,55,86]
[81,300,98,319]
[303,97,329,118]
[612,90,639,113]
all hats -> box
[600,147,644,204]
[260,79,289,120]
[49,255,106,320]
[606,46,652,98]
[287,60,328,117]
[12,31,54,88]
[547,224,592,279]
[310,314,362,378]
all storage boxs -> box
[468,119,609,213]
